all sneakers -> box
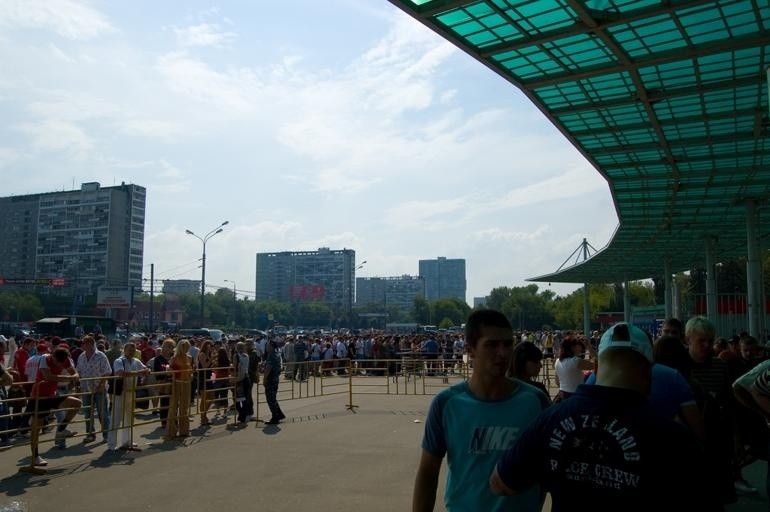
[85,436,94,442]
[103,437,108,443]
[56,430,73,438]
[33,454,47,466]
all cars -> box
[421,323,466,334]
[242,325,367,338]
[13,326,37,337]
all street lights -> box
[223,279,236,333]
[353,260,367,304]
[184,219,231,327]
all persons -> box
[412,308,770,512]
[280,332,466,379]
[0,331,286,466]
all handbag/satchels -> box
[193,371,203,379]
[135,388,148,408]
[156,378,171,394]
[213,383,224,389]
[106,375,123,396]
[251,371,260,383]
[233,377,250,394]
[320,353,324,360]
[7,385,27,407]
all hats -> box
[0,335,8,342]
[597,321,654,367]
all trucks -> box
[36,312,118,338]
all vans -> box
[179,328,223,339]
